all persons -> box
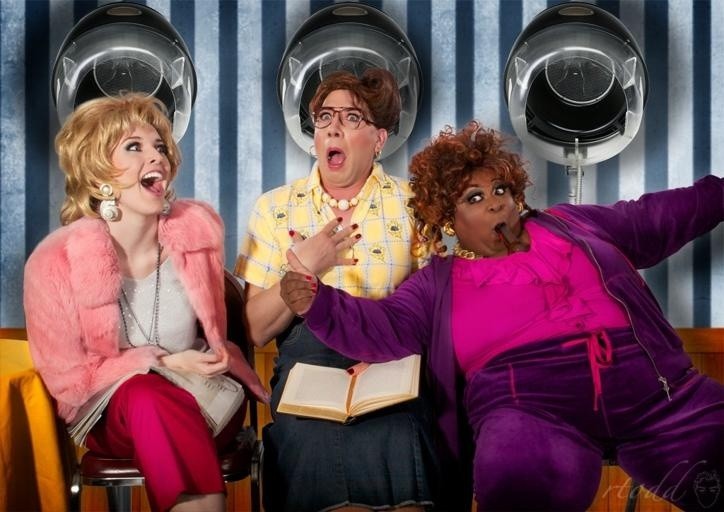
[20,91,272,512]
[279,121,724,511]
[233,65,465,510]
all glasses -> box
[309,104,376,129]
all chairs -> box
[69,267,262,510]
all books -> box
[274,352,423,423]
[63,365,245,449]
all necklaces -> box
[119,245,154,347]
[322,189,361,212]
[117,240,162,346]
[450,240,484,260]
